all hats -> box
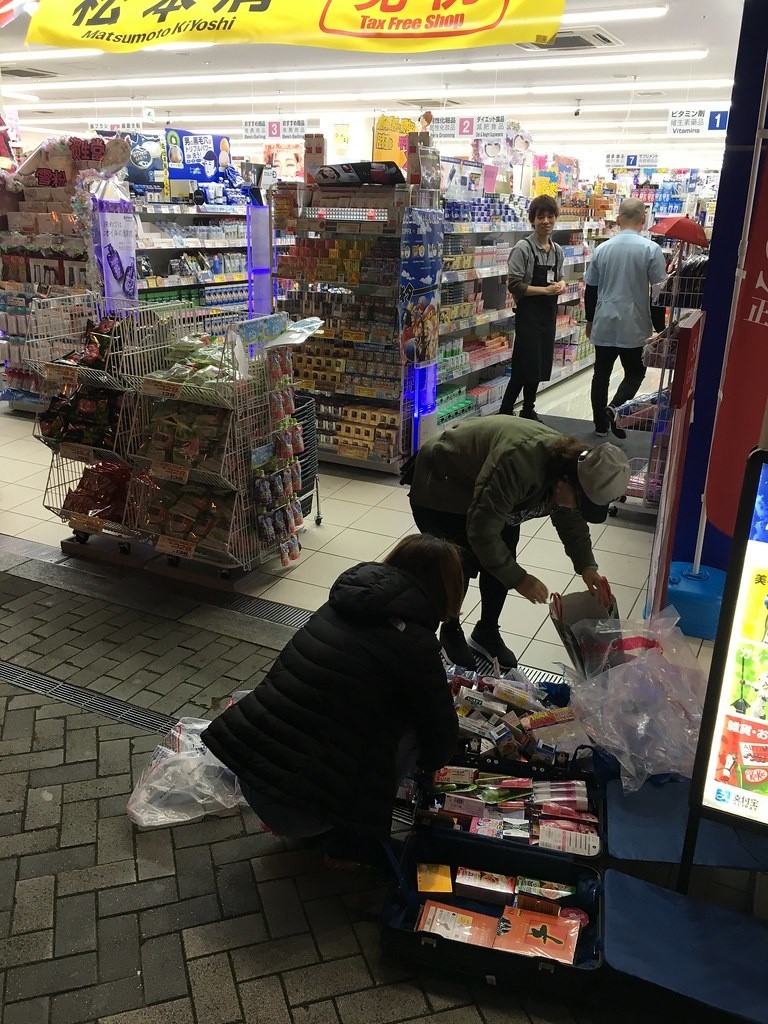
[577,442,631,524]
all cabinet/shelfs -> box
[0,167,131,418]
[437,178,596,435]
[275,175,436,473]
[131,181,275,338]
[607,253,709,518]
[586,184,721,332]
[31,291,318,573]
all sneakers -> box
[440,623,477,670]
[595,429,608,437]
[604,404,627,439]
[466,619,518,670]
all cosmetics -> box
[136,250,249,280]
[1,254,100,398]
[433,674,592,834]
[154,216,254,241]
[129,183,251,205]
[137,281,252,337]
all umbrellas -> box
[647,212,710,274]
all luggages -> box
[375,750,768,1024]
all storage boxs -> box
[447,682,617,785]
[379,831,605,1010]
[291,394,318,522]
[413,755,607,870]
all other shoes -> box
[324,833,383,874]
[498,410,516,416]
[520,411,543,423]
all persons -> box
[582,198,668,439]
[408,411,632,668]
[262,144,304,176]
[199,531,467,870]
[314,165,342,184]
[497,195,568,422]
[369,162,392,185]
